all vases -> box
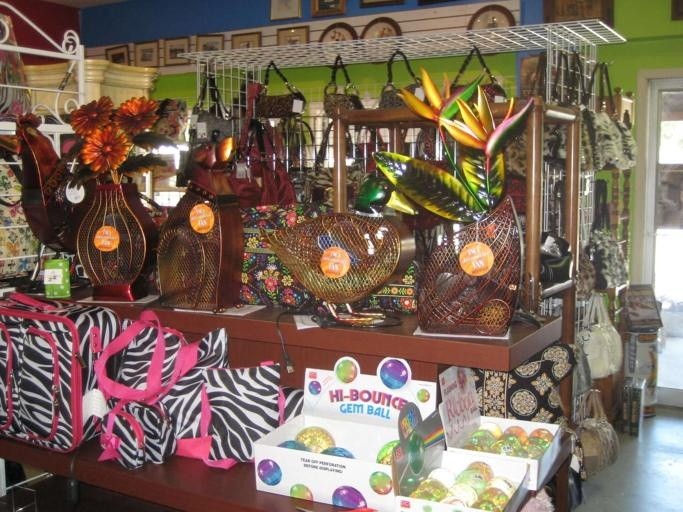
[155,161,243,311]
[76,185,147,301]
[416,194,523,338]
[258,215,401,327]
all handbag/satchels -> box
[578,295,623,379]
[578,389,618,474]
[0,293,304,470]
[189,49,428,207]
[586,179,628,286]
[0,155,41,279]
[544,52,637,170]
[451,48,506,104]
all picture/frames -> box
[134,41,158,67]
[310,0,346,15]
[542,0,611,25]
[195,33,224,51]
[231,31,262,48]
[163,37,190,64]
[104,44,130,65]
[359,0,403,6]
[275,25,308,46]
[269,0,300,20]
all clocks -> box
[467,4,515,55]
[370,66,534,222]
[360,16,402,64]
[318,22,358,69]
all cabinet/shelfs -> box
[596,86,635,426]
[331,95,582,512]
[0,1,83,282]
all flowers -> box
[68,98,159,185]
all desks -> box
[2,290,570,512]
